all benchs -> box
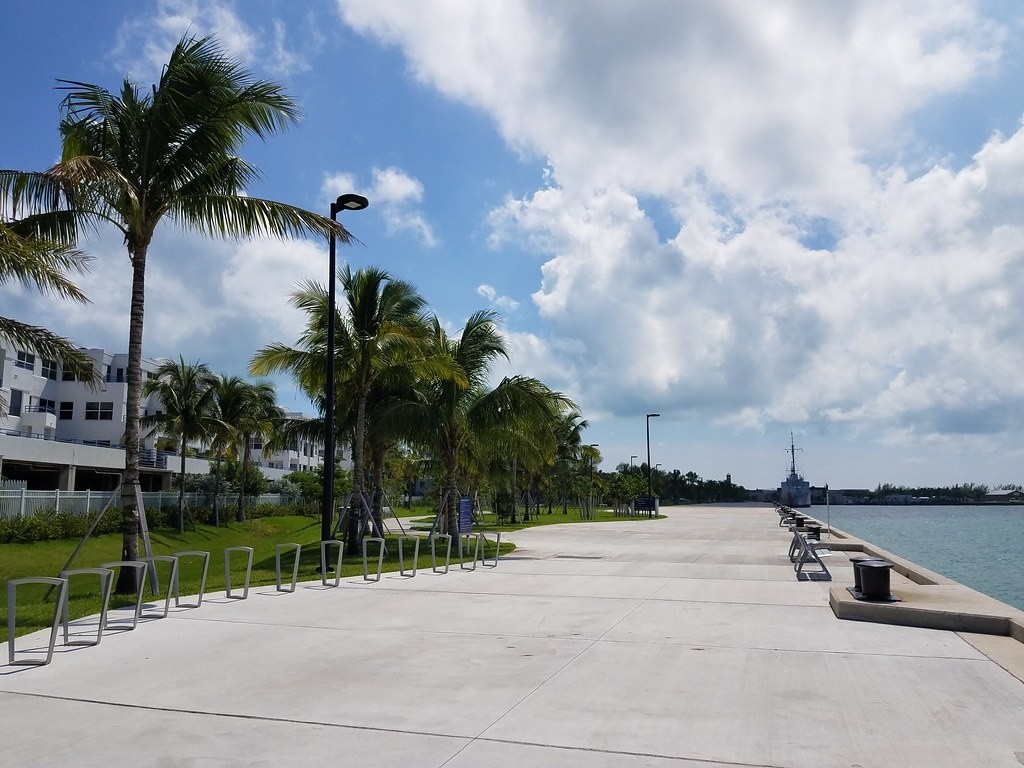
[772,501,833,582]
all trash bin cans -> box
[338,506,349,531]
